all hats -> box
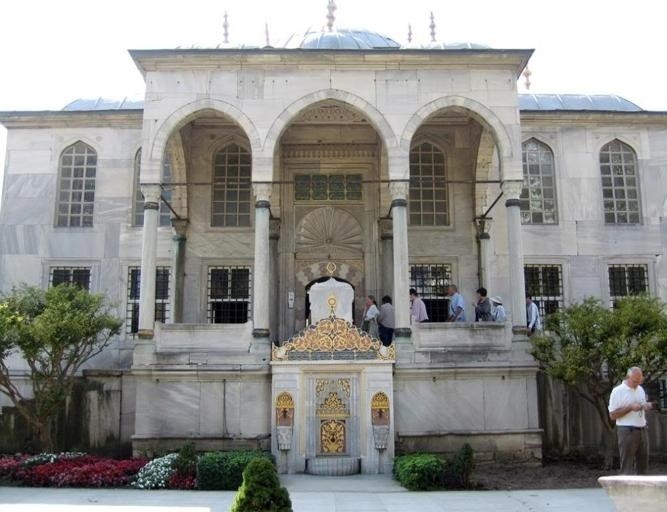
[490,296,503,304]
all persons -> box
[607,366,655,475]
[359,295,380,339]
[525,292,544,332]
[488,295,509,322]
[407,288,430,324]
[446,284,466,322]
[470,288,495,322]
[374,296,394,345]
